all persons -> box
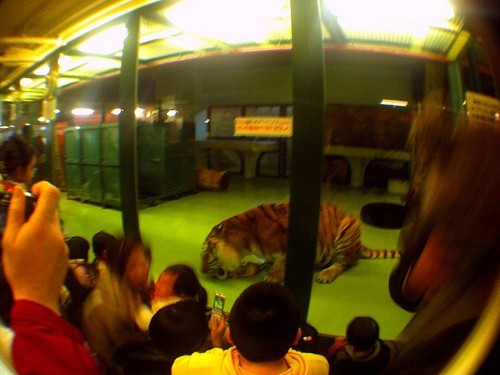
[82,231,226,375]
[329,317,396,375]
[171,281,329,375]
[0,134,100,375]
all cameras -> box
[0,191,36,237]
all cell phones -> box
[209,293,225,330]
